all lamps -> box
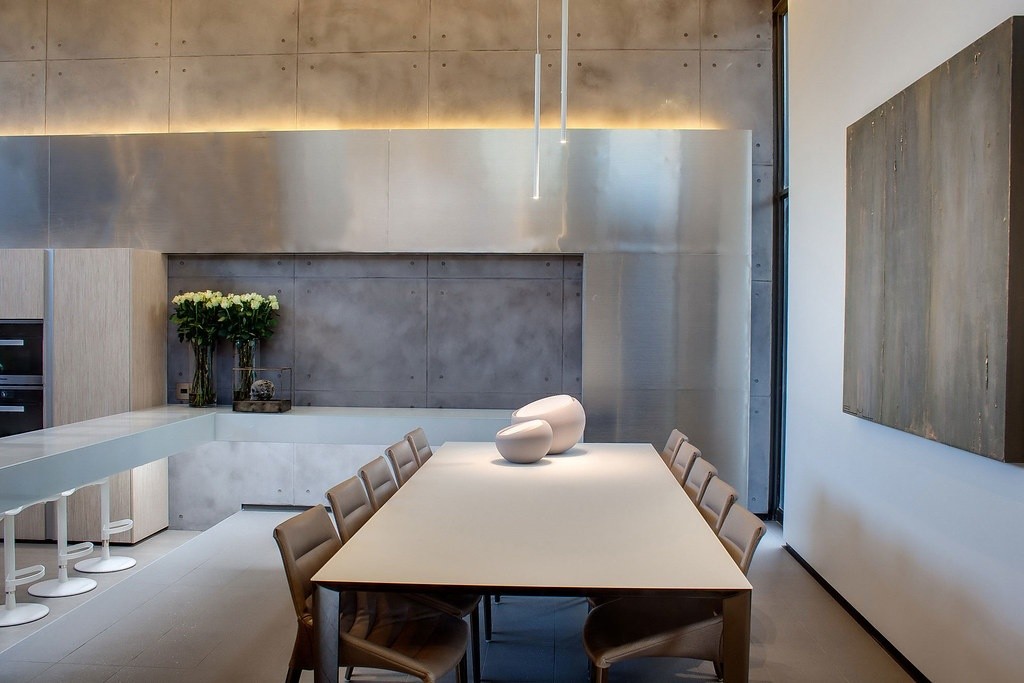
[532,0,571,199]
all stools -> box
[0,476,137,627]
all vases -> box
[232,339,258,399]
[189,337,217,407]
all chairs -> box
[326,475,492,683]
[358,456,501,604]
[272,504,472,683]
[659,428,692,467]
[670,441,702,486]
[404,427,433,467]
[682,456,719,507]
[582,501,769,683]
[384,439,421,489]
[586,476,741,612]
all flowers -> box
[170,290,280,405]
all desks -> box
[310,438,756,683]
